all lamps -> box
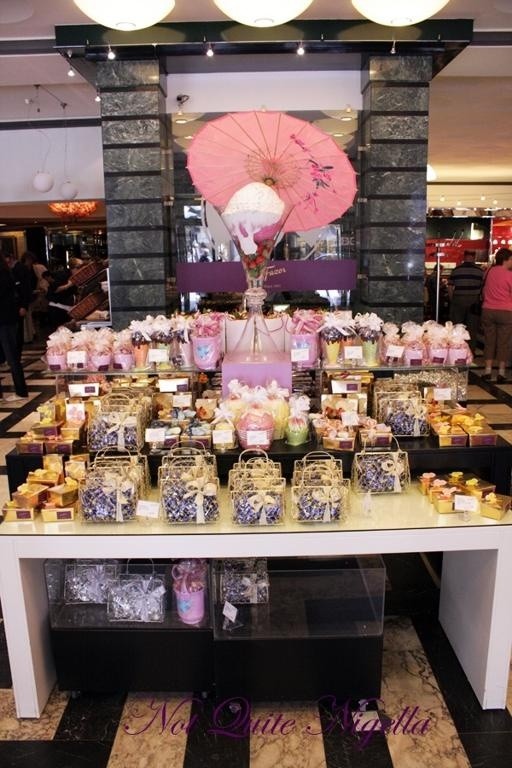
[206,43,214,57]
[173,94,190,124]
[71,0,176,32]
[24,81,79,202]
[105,38,116,60]
[391,40,397,54]
[46,201,98,223]
[351,0,450,28]
[296,40,305,56]
[212,0,316,28]
[67,67,100,102]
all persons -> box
[0,224,89,403]
[424,247,512,382]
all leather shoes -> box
[480,373,492,379]
[497,373,507,381]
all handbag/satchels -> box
[78,386,152,522]
[62,560,165,625]
[371,378,432,437]
[468,299,482,316]
[217,559,270,605]
[158,437,351,526]
[352,433,412,496]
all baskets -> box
[67,291,102,322]
[69,261,100,288]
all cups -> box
[289,331,319,370]
[173,562,208,626]
[320,324,466,367]
[188,332,223,371]
[42,329,189,372]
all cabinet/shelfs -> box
[81,267,113,330]
[0,365,512,720]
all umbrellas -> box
[184,111,359,233]
